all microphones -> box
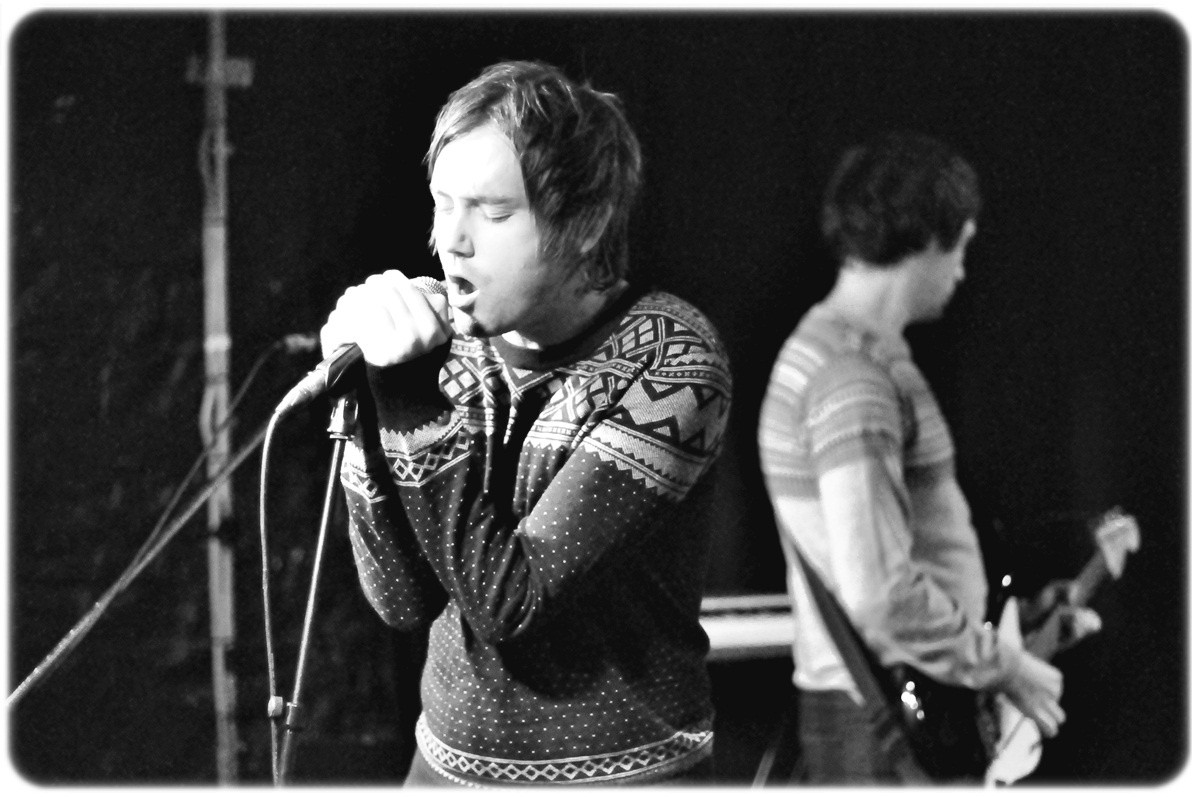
[276,276,448,417]
[281,333,323,357]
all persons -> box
[759,136,1099,784]
[321,60,733,785]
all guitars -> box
[901,505,1140,787]
